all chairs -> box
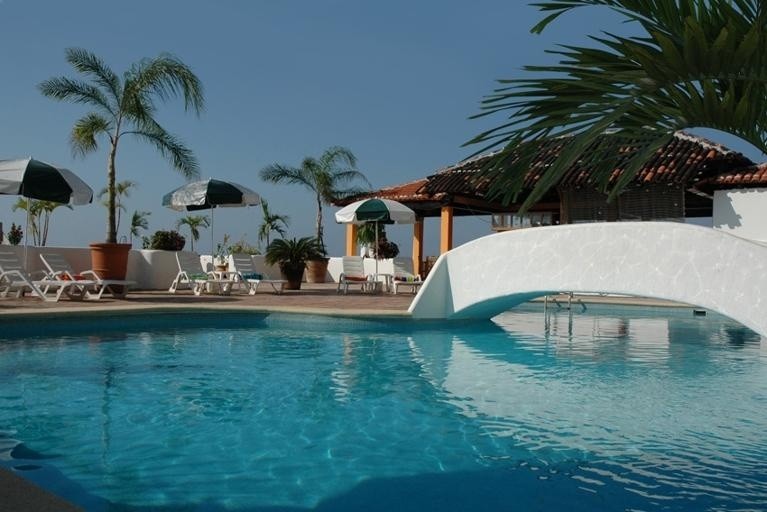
[168,251,289,296]
[337,256,424,295]
[0,250,136,302]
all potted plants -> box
[262,236,329,290]
[257,144,373,282]
[34,47,208,293]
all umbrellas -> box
[0,156,93,297]
[162,176,262,268]
[335,195,416,280]
